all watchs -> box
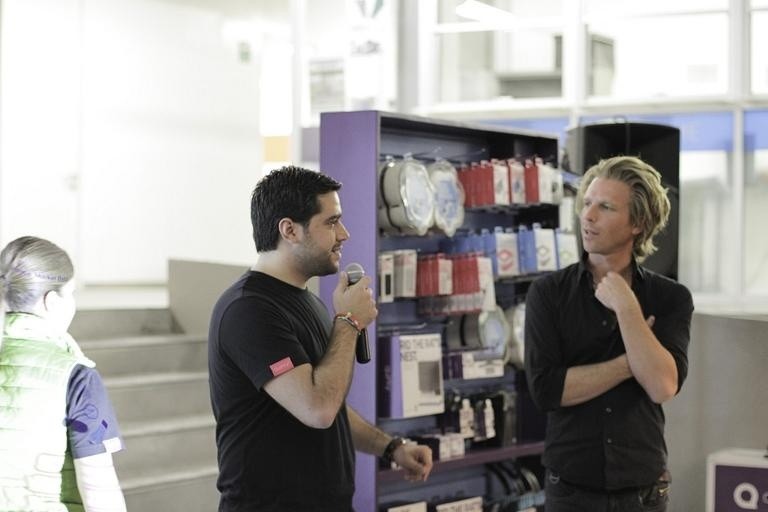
[381,435,406,467]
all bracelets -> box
[334,311,362,335]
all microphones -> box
[345,263,370,364]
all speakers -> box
[566,123,679,282]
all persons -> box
[2,236,129,511]
[206,165,433,510]
[524,154,694,511]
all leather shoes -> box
[545,464,674,494]
